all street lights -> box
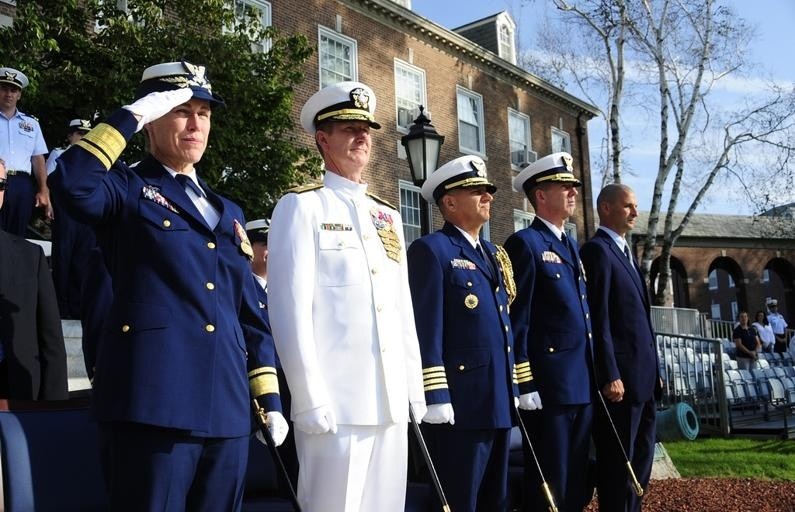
[402,103,445,238]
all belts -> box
[7,170,29,177]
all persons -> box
[264,80,428,512]
[407,156,524,510]
[42,112,91,177]
[732,297,794,370]
[577,182,664,512]
[0,161,68,413]
[499,151,602,510]
[238,209,270,329]
[0,63,56,239]
[50,57,291,512]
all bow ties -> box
[175,173,203,198]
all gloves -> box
[409,403,428,424]
[294,404,338,435]
[519,392,543,410]
[423,403,456,425]
[122,88,195,133]
[256,410,289,447]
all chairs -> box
[657,333,795,415]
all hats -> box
[766,299,778,306]
[421,155,498,208]
[68,119,92,131]
[513,151,582,194]
[243,219,271,241]
[300,81,381,138]
[0,67,29,91]
[135,61,226,111]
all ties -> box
[561,232,570,251]
[625,246,630,264]
[477,244,498,279]
